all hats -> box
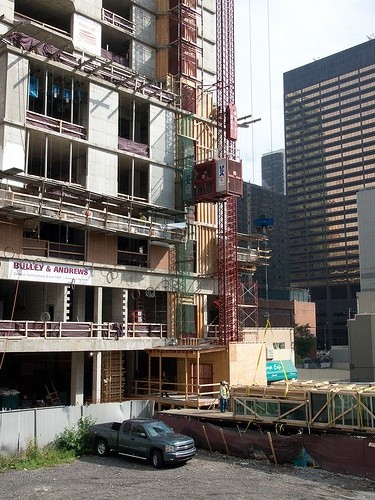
[222,381,227,385]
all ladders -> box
[45,381,61,406]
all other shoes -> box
[225,409,228,412]
[222,411,225,413]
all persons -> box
[139,212,146,225]
[220,381,229,413]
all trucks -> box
[88,418,197,469]
